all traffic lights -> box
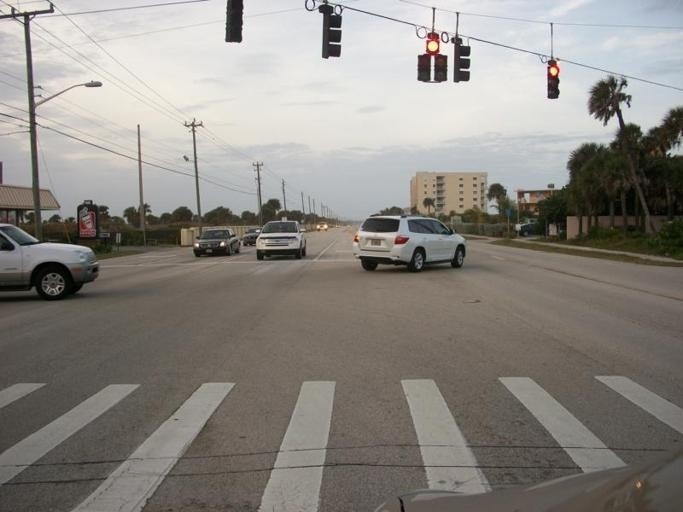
[426,39,442,54]
[433,54,447,84]
[321,14,342,58]
[417,53,430,83]
[452,44,470,83]
[545,60,559,100]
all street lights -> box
[182,156,203,235]
[26,78,102,239]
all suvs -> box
[352,213,466,272]
[0,219,99,300]
[255,219,305,260]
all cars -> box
[315,222,327,232]
[192,226,239,257]
[513,216,543,236]
[243,227,261,246]
